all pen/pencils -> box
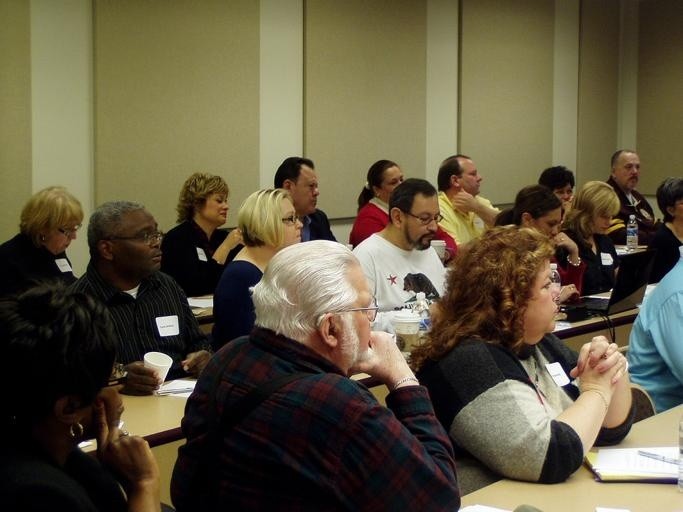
[157,388,194,396]
[638,450,681,466]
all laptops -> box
[567,251,649,315]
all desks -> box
[78,243,683,512]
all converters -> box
[568,307,588,321]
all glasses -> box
[114,232,163,243]
[58,224,82,236]
[282,215,297,223]
[407,212,444,225]
[336,297,379,322]
[105,364,129,387]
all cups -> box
[429,238,446,268]
[141,350,174,397]
[388,309,420,352]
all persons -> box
[159,149,682,497]
[0,185,216,512]
[170,240,461,511]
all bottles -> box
[547,262,559,307]
[624,213,638,252]
[412,292,431,335]
